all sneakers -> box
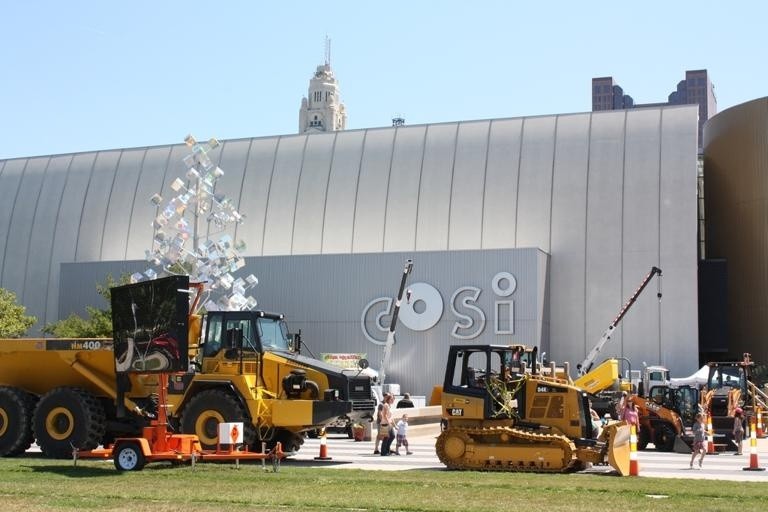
[374,450,413,456]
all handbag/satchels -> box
[377,425,389,440]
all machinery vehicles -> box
[0,274,379,474]
[435,266,756,472]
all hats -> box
[736,408,743,414]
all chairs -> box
[466,366,487,390]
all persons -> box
[587,399,600,438]
[373,391,414,456]
[621,398,640,460]
[690,413,710,467]
[733,408,744,455]
